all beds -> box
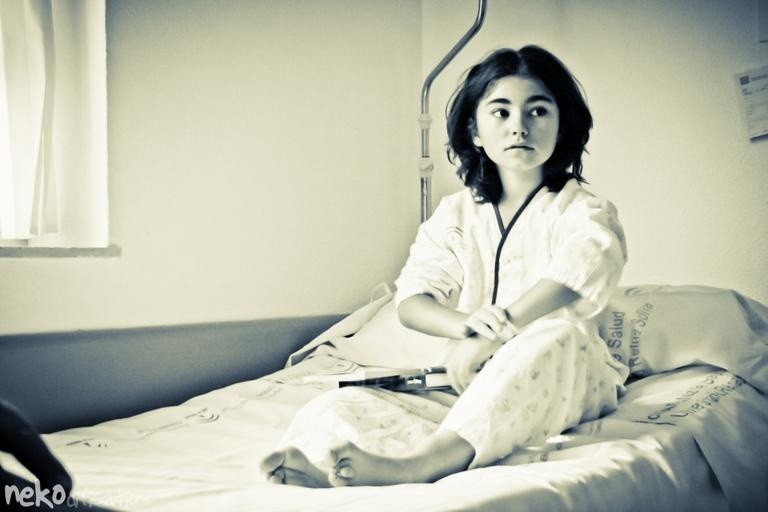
[1,288,768,510]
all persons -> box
[257,44,631,490]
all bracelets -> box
[492,320,518,346]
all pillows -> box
[349,289,745,373]
